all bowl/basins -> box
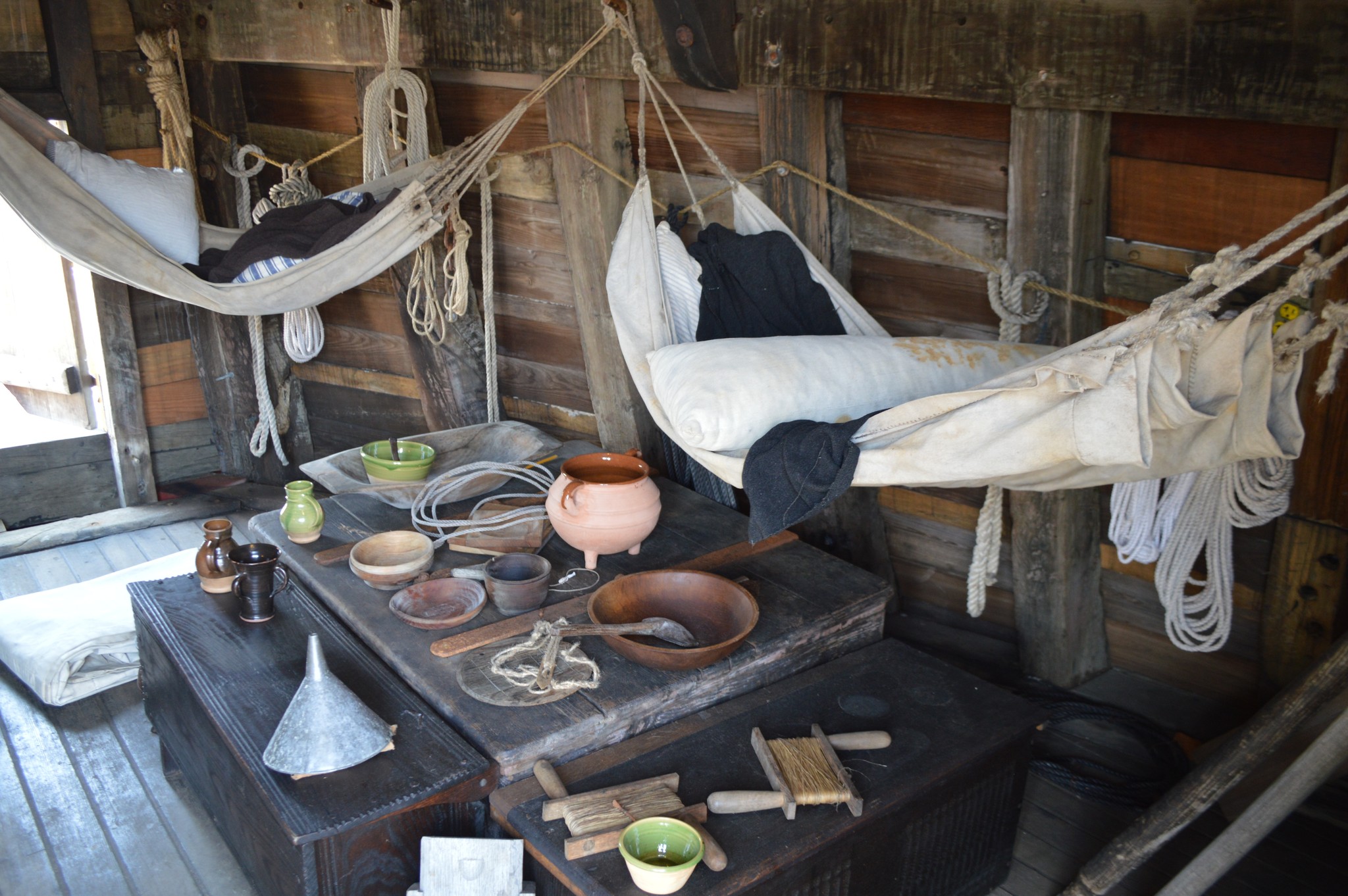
[484,552,552,617]
[618,817,706,893]
[360,440,438,485]
[348,530,434,590]
[586,569,759,670]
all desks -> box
[244,440,893,788]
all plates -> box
[388,578,489,629]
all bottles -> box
[279,480,324,545]
[195,518,244,595]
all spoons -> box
[550,617,699,647]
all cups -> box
[226,542,288,623]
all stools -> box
[483,632,1036,896]
[127,564,493,896]
